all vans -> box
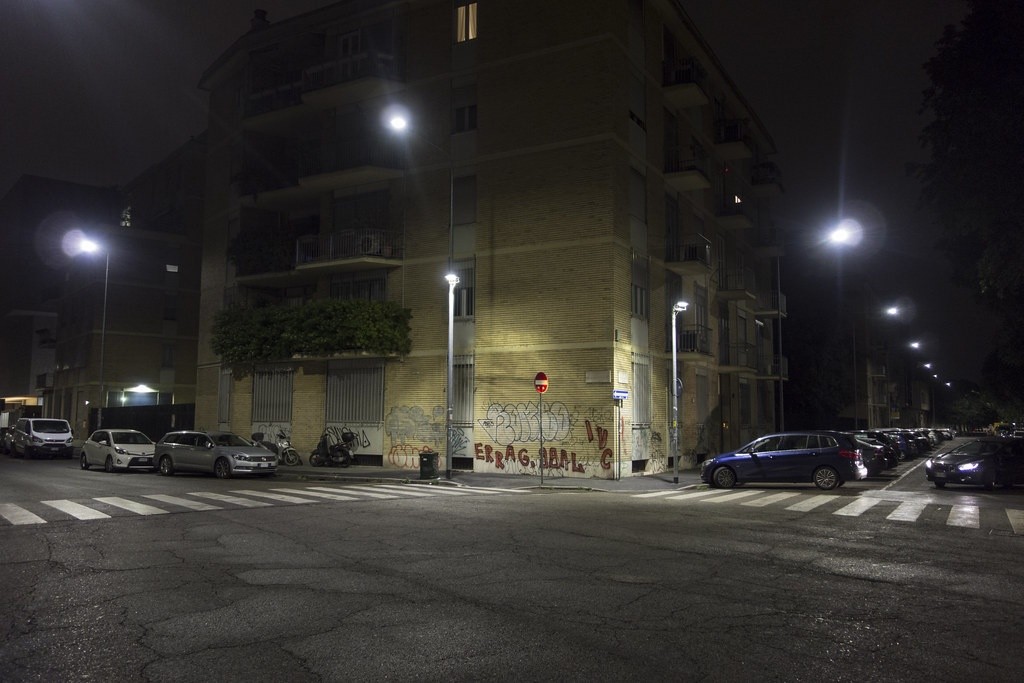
[9,417,75,460]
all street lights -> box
[774,230,852,436]
[671,301,693,484]
[386,113,456,270]
[441,273,460,477]
[79,240,110,426]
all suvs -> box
[698,430,867,490]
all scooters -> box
[309,431,356,468]
[250,428,300,466]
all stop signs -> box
[532,372,549,394]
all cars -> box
[1013,430,1024,440]
[840,427,957,478]
[79,428,159,473]
[924,435,1024,488]
[151,429,279,479]
[0,424,15,455]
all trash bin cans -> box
[419,451,439,480]
